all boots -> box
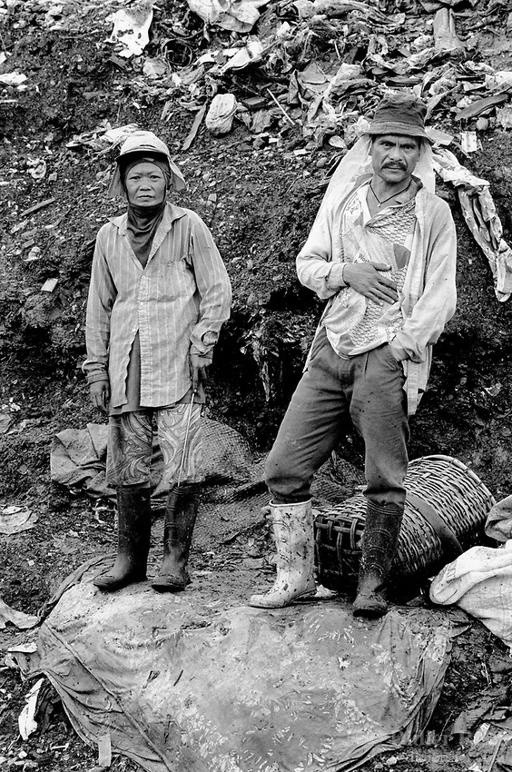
[351,500,403,620]
[248,497,318,610]
[93,480,154,586]
[152,483,201,592]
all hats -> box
[115,147,170,172]
[364,93,436,146]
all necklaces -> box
[369,177,413,207]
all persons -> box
[247,94,456,618]
[80,130,234,589]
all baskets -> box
[311,451,496,597]
